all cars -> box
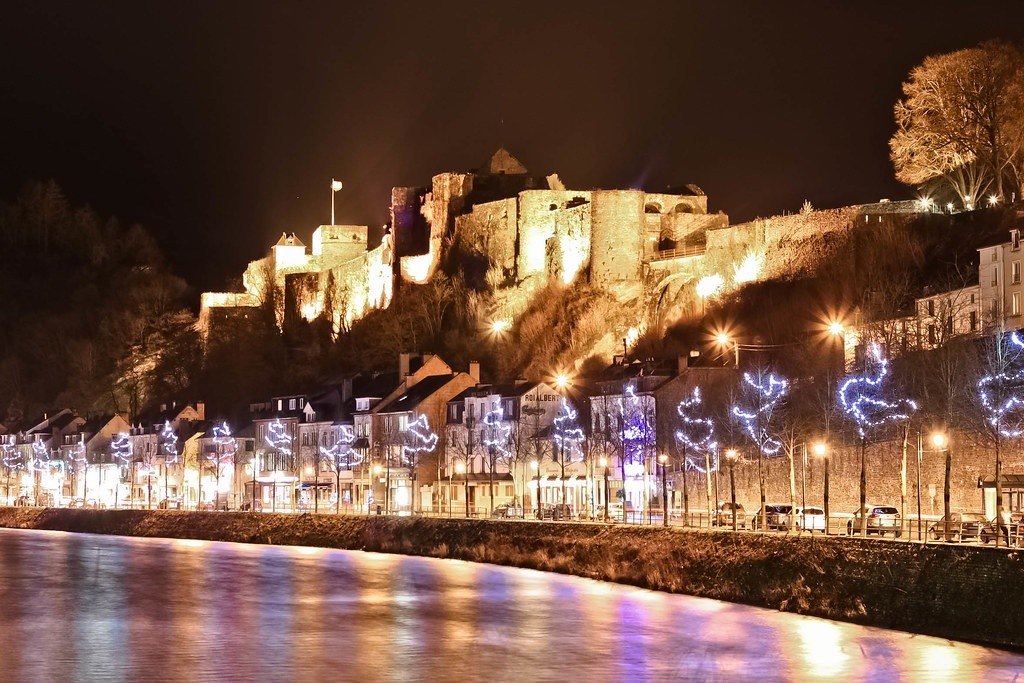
[241,501,265,515]
[927,512,989,542]
[980,514,1024,544]
[847,505,905,538]
[551,503,573,519]
[162,499,183,512]
[747,503,792,531]
[782,507,828,533]
[707,500,749,528]
[13,496,35,505]
[533,503,551,519]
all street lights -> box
[306,466,316,514]
[599,457,610,522]
[373,464,385,515]
[663,454,678,531]
[456,462,469,519]
[813,442,830,536]
[530,461,541,519]
[724,448,747,538]
[926,433,948,531]
